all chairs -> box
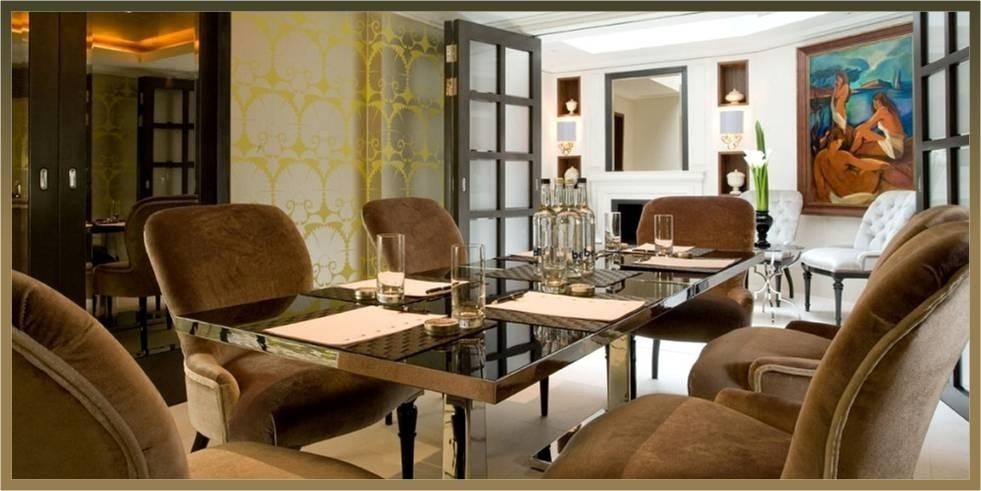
[687,205,971,401]
[94,195,199,329]
[739,189,804,307]
[634,195,755,396]
[361,197,550,416]
[143,202,418,480]
[13,268,381,480]
[799,190,917,326]
[539,218,970,479]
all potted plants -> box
[742,120,773,249]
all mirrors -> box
[604,65,689,171]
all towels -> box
[335,275,469,297]
[260,305,448,346]
[485,290,647,323]
[510,248,572,262]
[632,256,742,269]
[632,241,695,253]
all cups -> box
[450,242,486,330]
[654,214,674,258]
[376,233,406,306]
[605,212,622,253]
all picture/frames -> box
[795,23,913,216]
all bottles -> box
[14,180,22,198]
[532,177,597,294]
